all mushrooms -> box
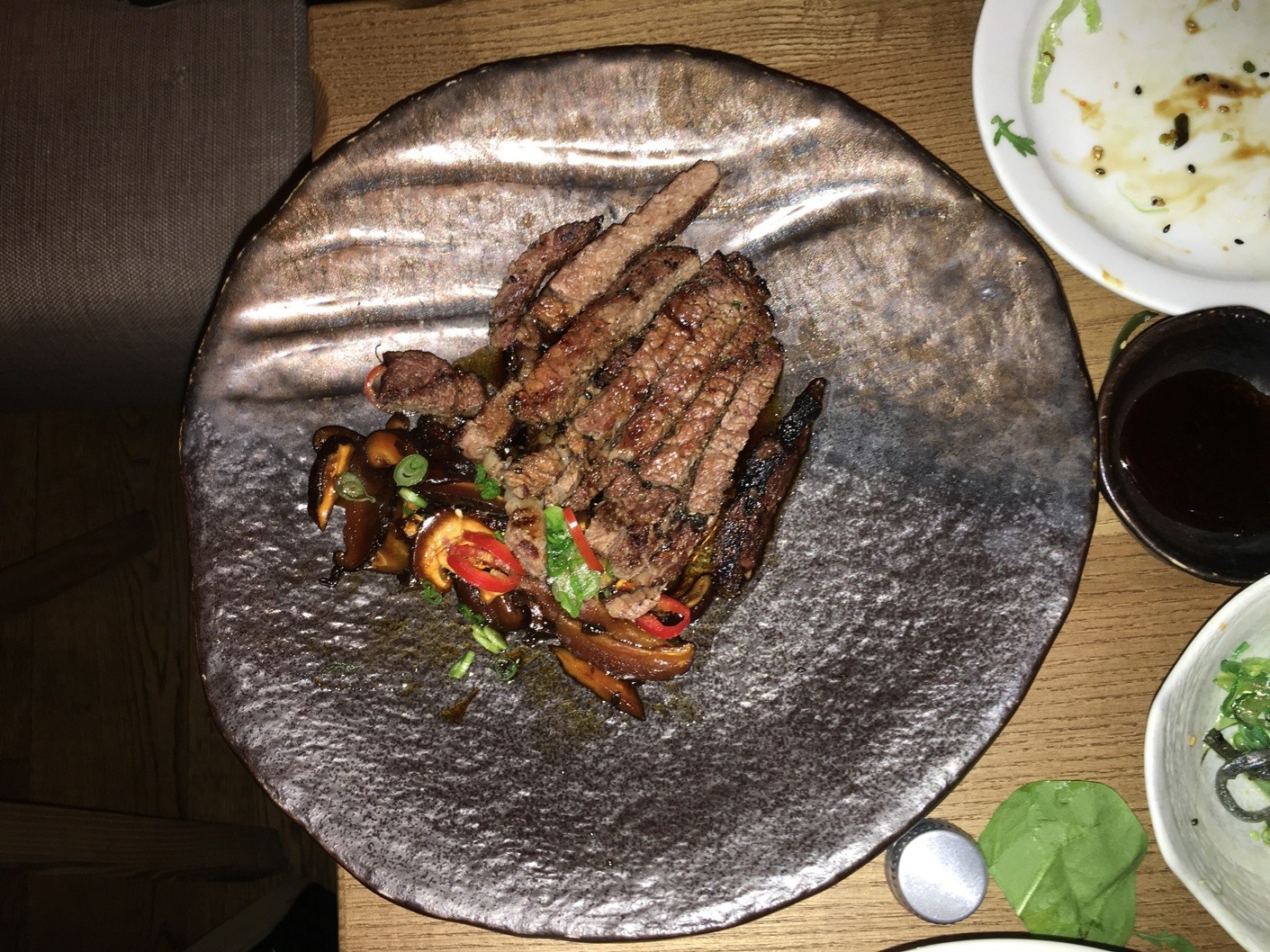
[308,412,697,721]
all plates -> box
[966,1,1270,316]
[177,38,1099,945]
[1143,571,1270,952]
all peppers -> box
[449,529,525,593]
[563,510,602,572]
[636,594,693,637]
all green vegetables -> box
[974,777,1149,950]
[1201,641,1270,840]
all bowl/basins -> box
[1095,306,1268,589]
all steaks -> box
[375,159,826,600]
